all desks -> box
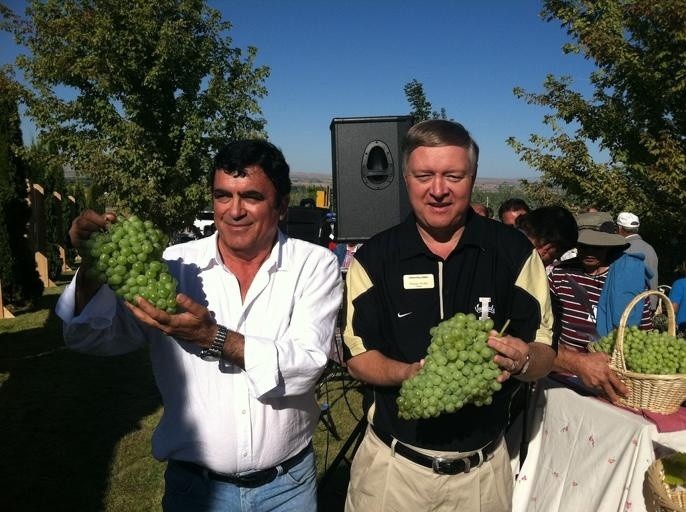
[513,374,686,512]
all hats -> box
[616,211,641,228]
[574,212,631,251]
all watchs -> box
[510,353,532,378]
[198,323,227,363]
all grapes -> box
[78,214,181,315]
[590,326,686,376]
[395,312,512,421]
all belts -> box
[370,424,505,475]
[167,438,314,488]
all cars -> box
[179,211,215,239]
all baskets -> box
[586,289,686,415]
[645,452,685,512]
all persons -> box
[336,114,560,511]
[469,198,686,402]
[299,199,315,207]
[51,136,345,511]
[329,240,365,278]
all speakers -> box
[329,116,414,243]
